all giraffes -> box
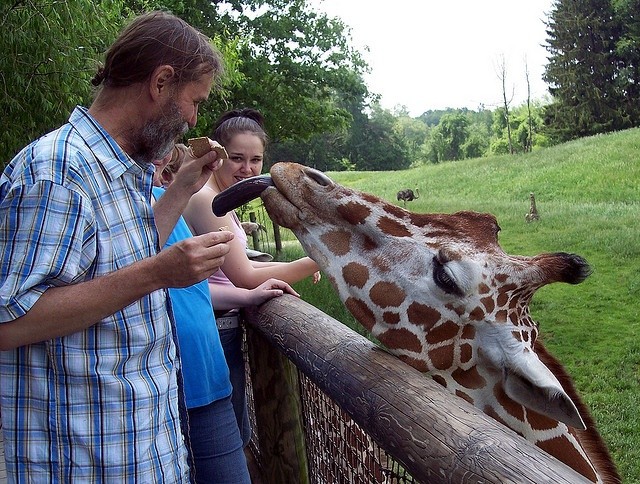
[210,160,621,483]
[239,321,403,483]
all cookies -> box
[212,145,228,160]
[187,136,212,158]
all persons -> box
[181,107,321,451]
[149,138,302,484]
[160,143,188,190]
[1,10,236,484]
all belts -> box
[217,317,238,330]
